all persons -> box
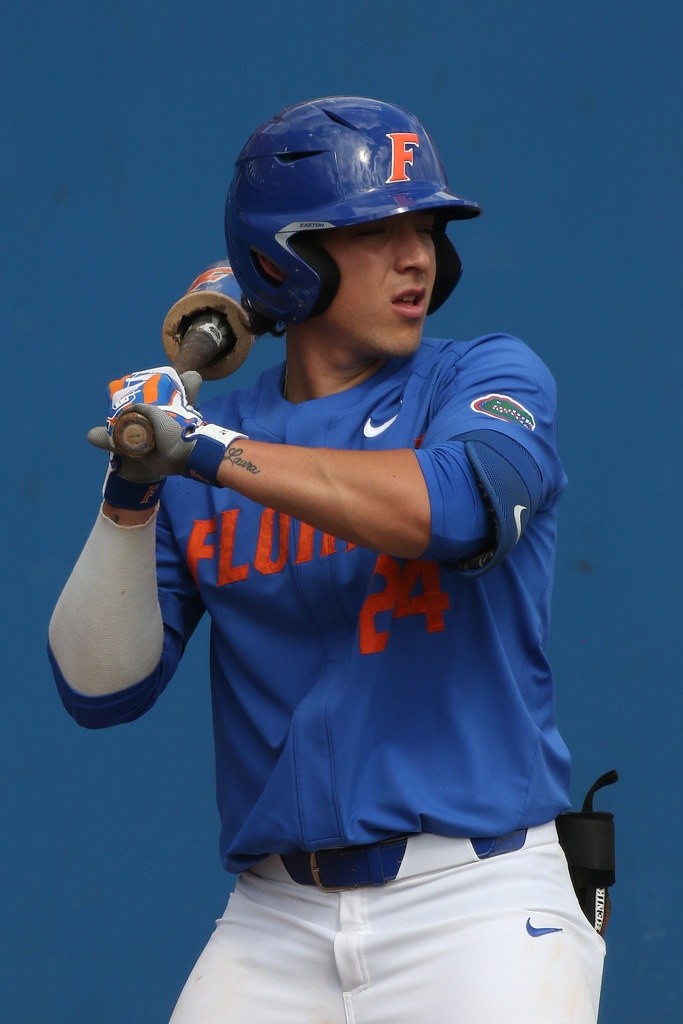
[43,93,622,1024]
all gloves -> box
[87,366,249,511]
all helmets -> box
[224,95,483,324]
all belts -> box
[279,828,528,893]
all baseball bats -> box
[114,256,255,462]
